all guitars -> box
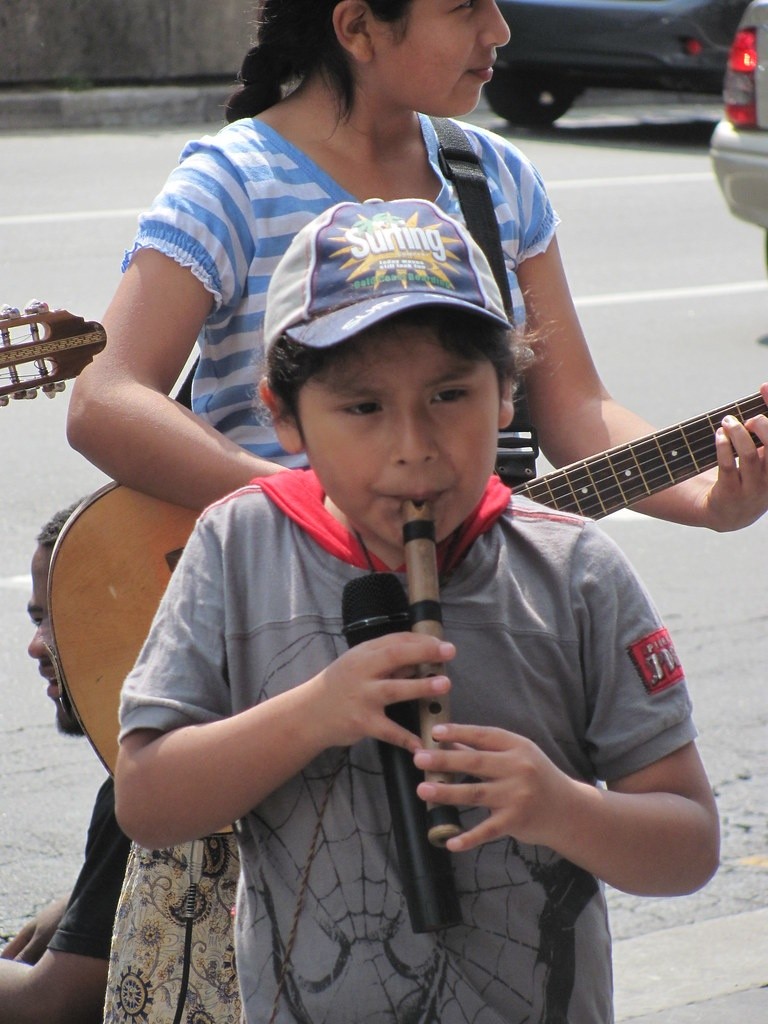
[1,302,107,405]
[32,387,768,841]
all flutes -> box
[402,497,461,851]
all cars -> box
[477,0,752,124]
[715,0,768,231]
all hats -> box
[261,197,513,374]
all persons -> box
[112,197,719,1024]
[0,498,134,1024]
[67,0,768,1024]
